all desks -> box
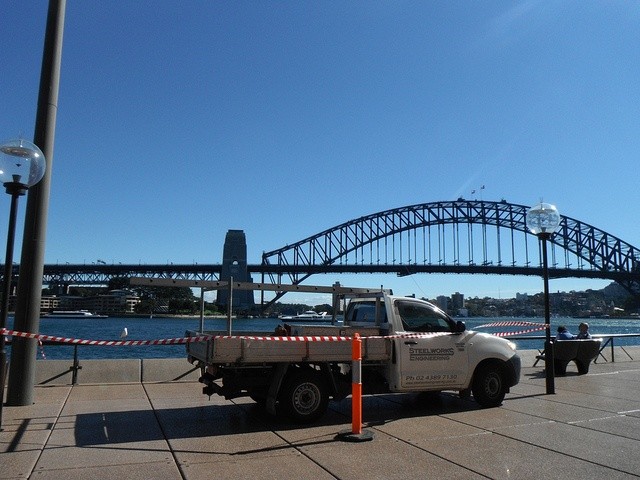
[591,332,639,363]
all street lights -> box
[526,202,561,394]
[0,139,47,431]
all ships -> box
[280,310,337,322]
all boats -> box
[42,310,109,319]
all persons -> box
[577,323,591,339]
[554,325,574,339]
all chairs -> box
[535,339,601,378]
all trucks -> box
[184,291,520,419]
